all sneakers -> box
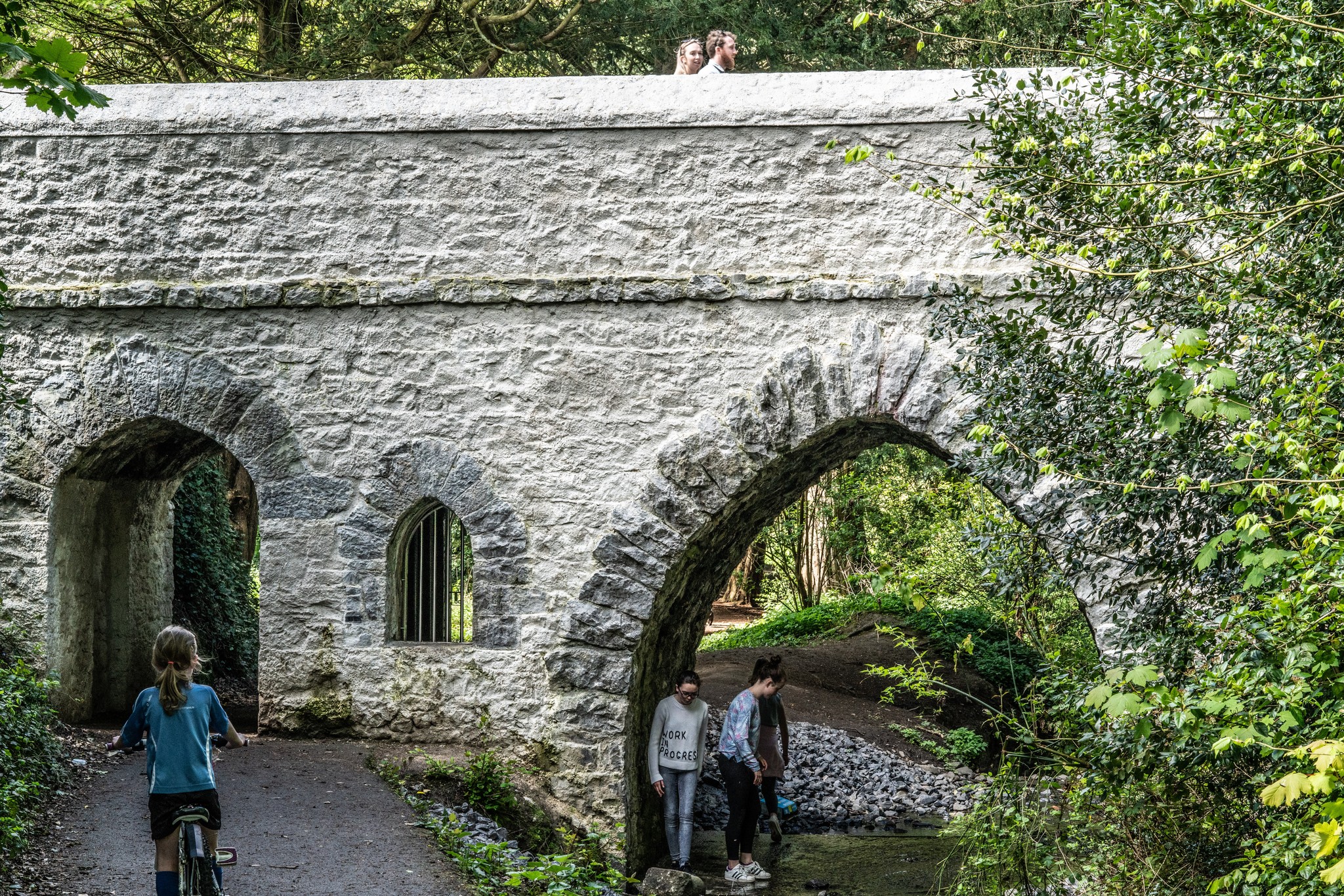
[670,861,696,876]
[741,864,771,879]
[754,816,782,842]
[724,864,755,882]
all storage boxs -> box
[759,794,797,821]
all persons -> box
[648,671,708,872]
[671,39,705,76]
[746,657,791,844]
[716,658,789,885]
[109,623,251,896]
[696,28,739,75]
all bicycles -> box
[106,732,253,896]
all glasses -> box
[678,686,700,698]
